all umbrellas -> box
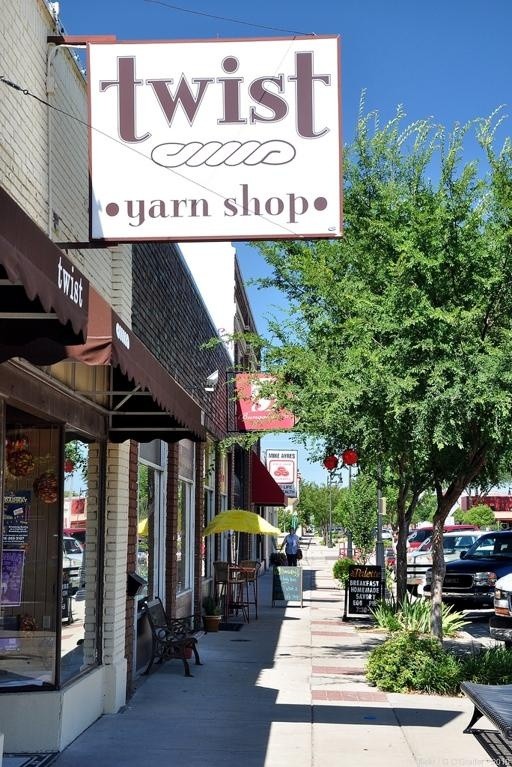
[202,507,281,614]
[138,511,181,535]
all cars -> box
[374,527,394,541]
[63,536,84,570]
[404,525,512,651]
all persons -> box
[280,526,300,565]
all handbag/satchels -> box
[296,549,303,559]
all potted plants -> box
[202,596,223,632]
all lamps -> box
[33,425,59,504]
[7,423,36,478]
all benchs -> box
[141,597,204,677]
[461,626,512,767]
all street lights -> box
[342,448,358,559]
[323,456,337,548]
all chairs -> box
[235,560,261,620]
[65,540,74,553]
[213,560,250,625]
[502,543,512,552]
[443,537,473,550]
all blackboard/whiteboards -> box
[349,565,381,614]
[272,566,303,601]
